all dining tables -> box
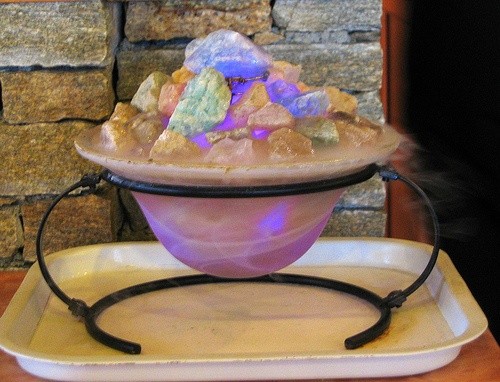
[0,267,500,382]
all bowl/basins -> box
[78,124,402,281]
